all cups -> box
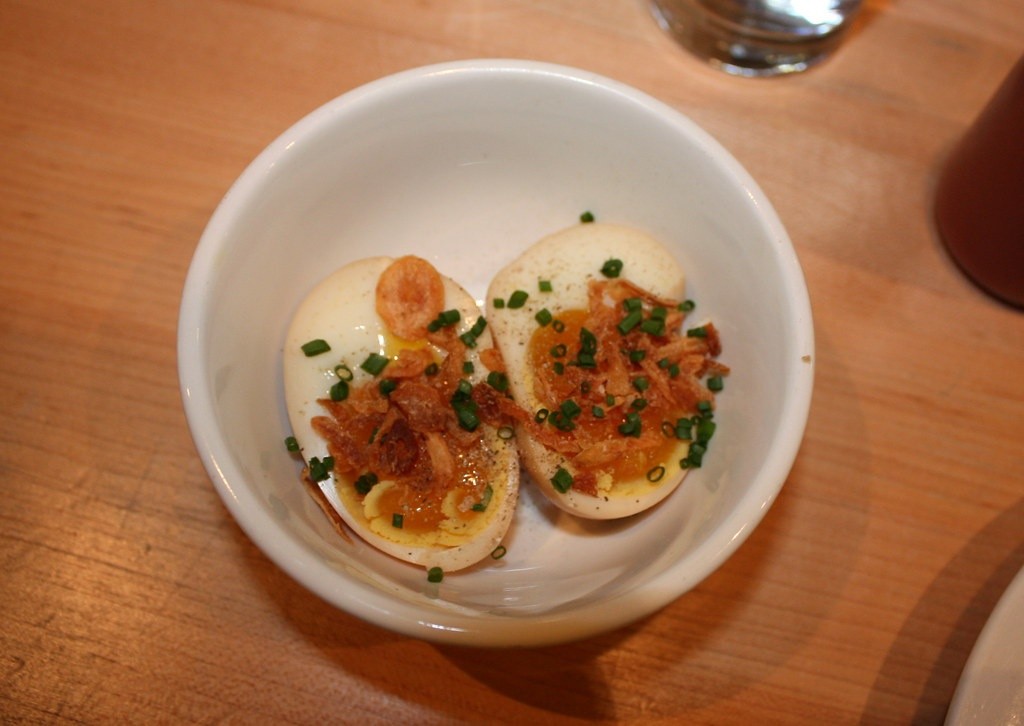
[647,0,860,78]
[936,52,1024,311]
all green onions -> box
[282,211,723,584]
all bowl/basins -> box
[176,57,814,647]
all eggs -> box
[284,254,522,574]
[487,221,698,520]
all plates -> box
[942,565,1024,726]
